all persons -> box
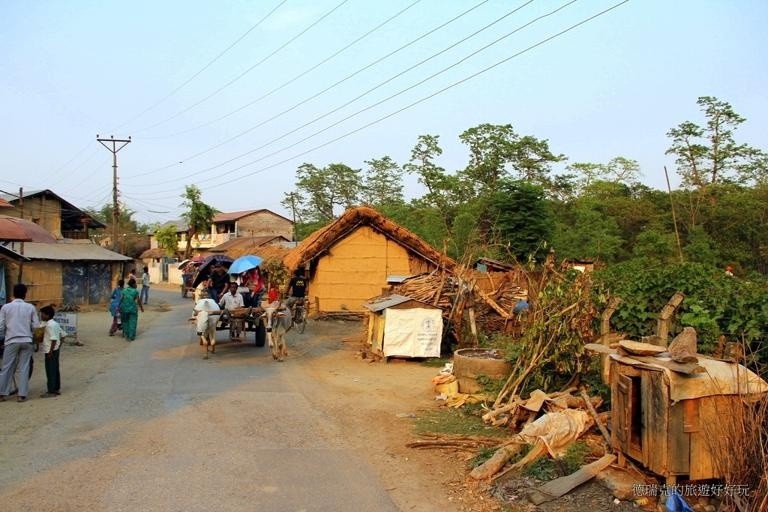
[138,266,150,304]
[40,306,66,398]
[119,279,144,341]
[108,280,126,336]
[194,263,267,341]
[129,269,137,282]
[285,270,309,318]
[0,284,41,402]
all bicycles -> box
[278,289,308,334]
[0,345,34,395]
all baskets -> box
[30,326,47,343]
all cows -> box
[188,298,221,361]
[258,301,292,363]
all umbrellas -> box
[178,255,264,288]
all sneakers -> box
[16,395,28,402]
[40,392,56,398]
[0,395,7,402]
